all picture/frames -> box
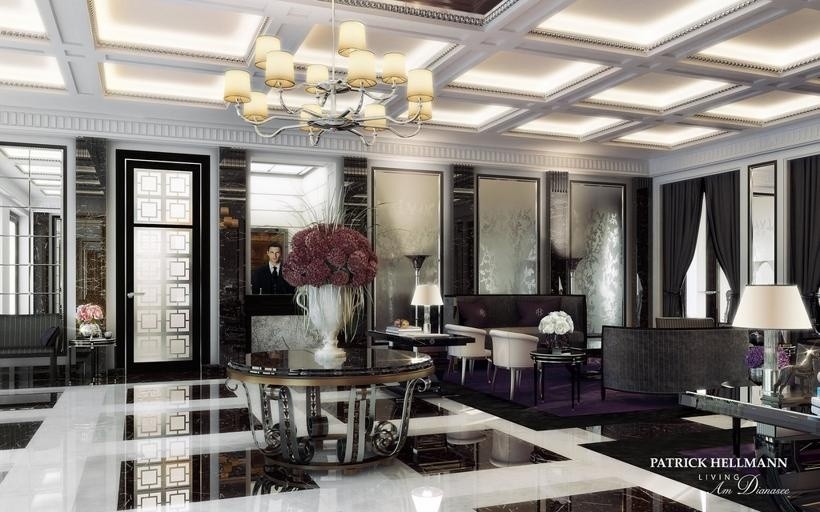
[250,228,289,294]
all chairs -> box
[447,428,533,468]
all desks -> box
[69,337,119,385]
[227,349,432,467]
[368,329,476,393]
[679,385,820,512]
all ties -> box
[272,267,278,276]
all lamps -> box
[223,0,432,146]
[218,207,239,231]
[404,254,444,333]
[410,486,444,512]
[731,284,813,401]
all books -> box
[385,324,423,335]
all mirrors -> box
[748,162,777,287]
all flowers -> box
[283,184,407,340]
[76,303,103,324]
[745,345,790,367]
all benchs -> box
[0,313,65,381]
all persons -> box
[252,243,294,292]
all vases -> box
[750,368,792,386]
[80,323,100,337]
[297,282,354,362]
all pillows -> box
[41,327,59,345]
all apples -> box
[394,318,409,329]
